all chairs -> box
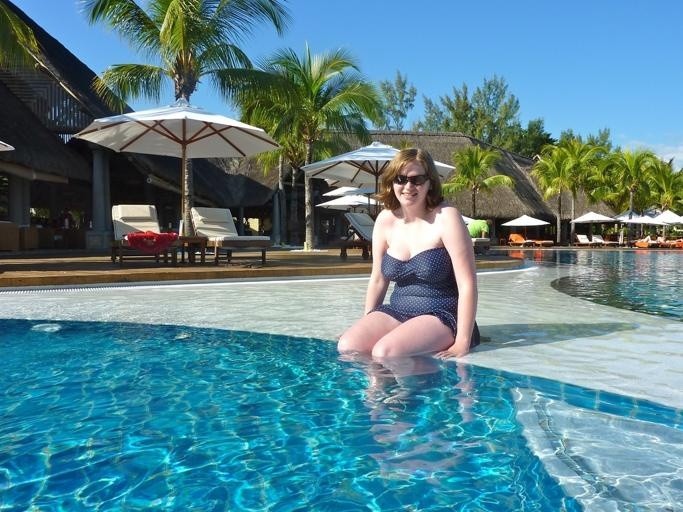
[508,234,683,249]
[471,238,490,261]
[190,206,270,266]
[344,212,375,260]
[111,204,180,266]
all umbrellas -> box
[500,215,551,241]
[300,141,456,249]
[74,97,281,261]
[569,206,683,226]
[0,141,16,152]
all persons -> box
[468,219,493,239]
[336,147,478,358]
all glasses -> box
[393,174,430,186]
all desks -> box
[164,236,209,265]
